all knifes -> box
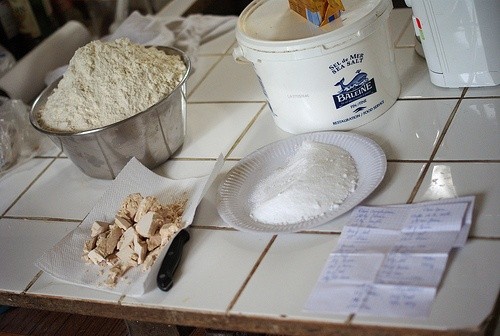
[156,216,191,292]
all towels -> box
[106,10,238,61]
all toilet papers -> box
[0,20,90,104]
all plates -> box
[216,131,388,234]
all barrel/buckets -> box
[232,0,401,134]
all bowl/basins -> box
[29,44,191,179]
